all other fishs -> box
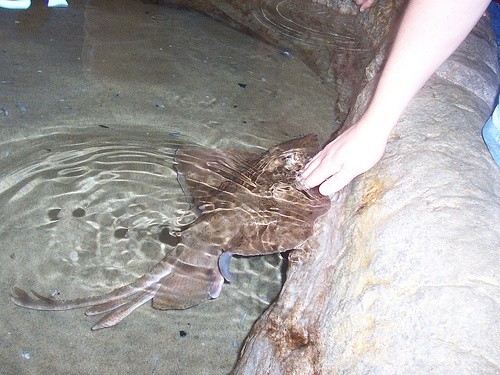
[8,132,331,333]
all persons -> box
[353,0,374,12]
[294,0,494,196]
